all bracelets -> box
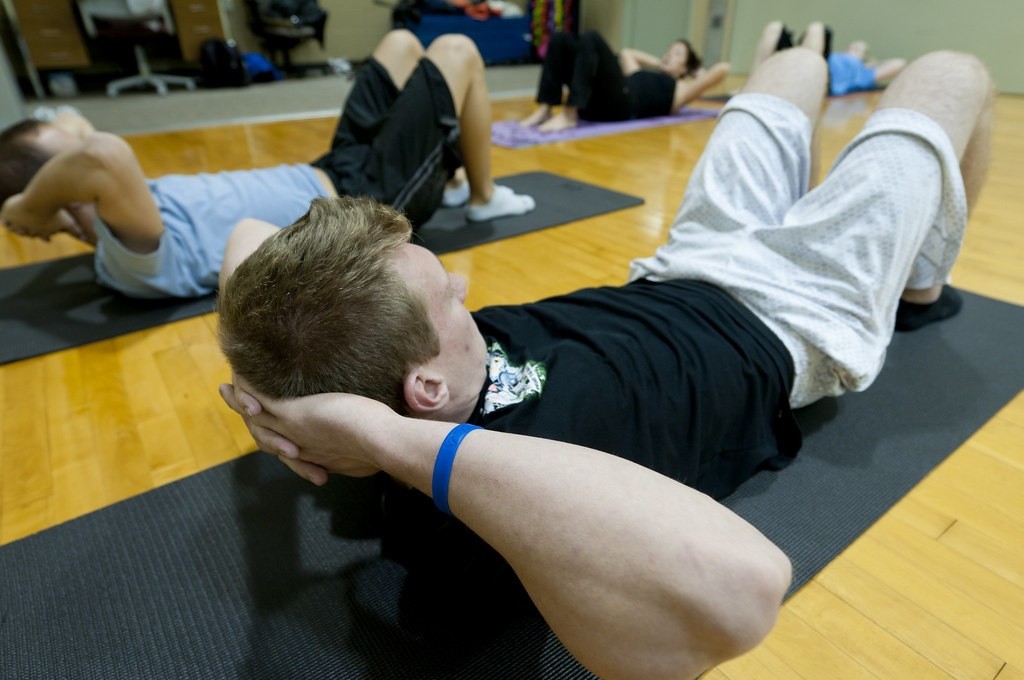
[430,424,485,517]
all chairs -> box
[78,0,196,98]
[240,0,330,78]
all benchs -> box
[393,0,532,65]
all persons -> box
[0,28,537,301]
[728,20,907,97]
[217,46,998,680]
[521,28,731,133]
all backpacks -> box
[197,35,247,89]
